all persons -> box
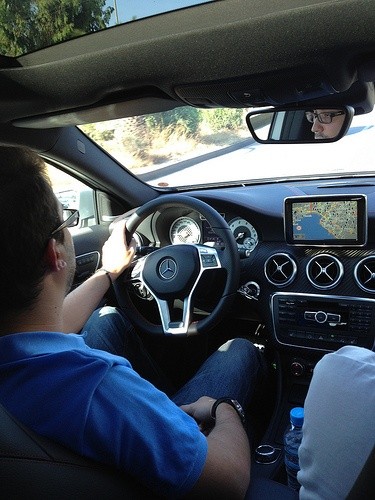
[311,109,345,139]
[0,145,273,500]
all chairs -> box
[0,402,154,500]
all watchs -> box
[212,397,245,424]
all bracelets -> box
[96,269,113,286]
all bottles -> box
[284,406,305,491]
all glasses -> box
[49,209,80,237]
[306,110,345,124]
